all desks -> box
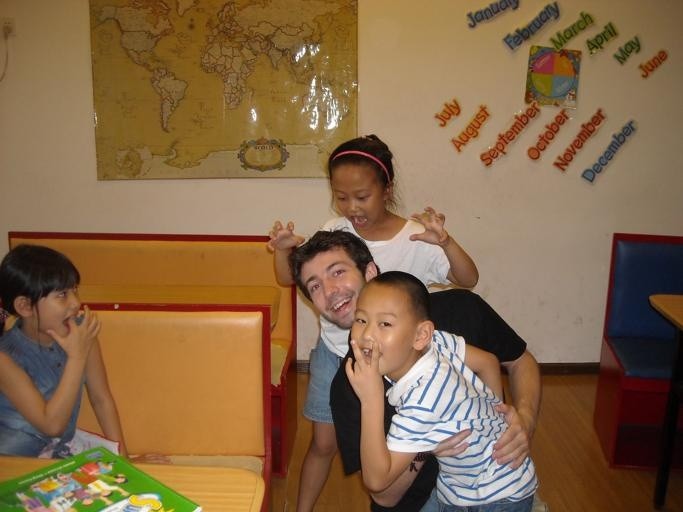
[1,458,267,511]
[77,285,278,326]
[649,294,683,509]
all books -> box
[0,444,202,512]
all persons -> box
[0,241,175,465]
[345,270,541,512]
[287,225,549,512]
[266,133,480,512]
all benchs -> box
[5,302,273,480]
[593,230,683,472]
[8,232,298,477]
